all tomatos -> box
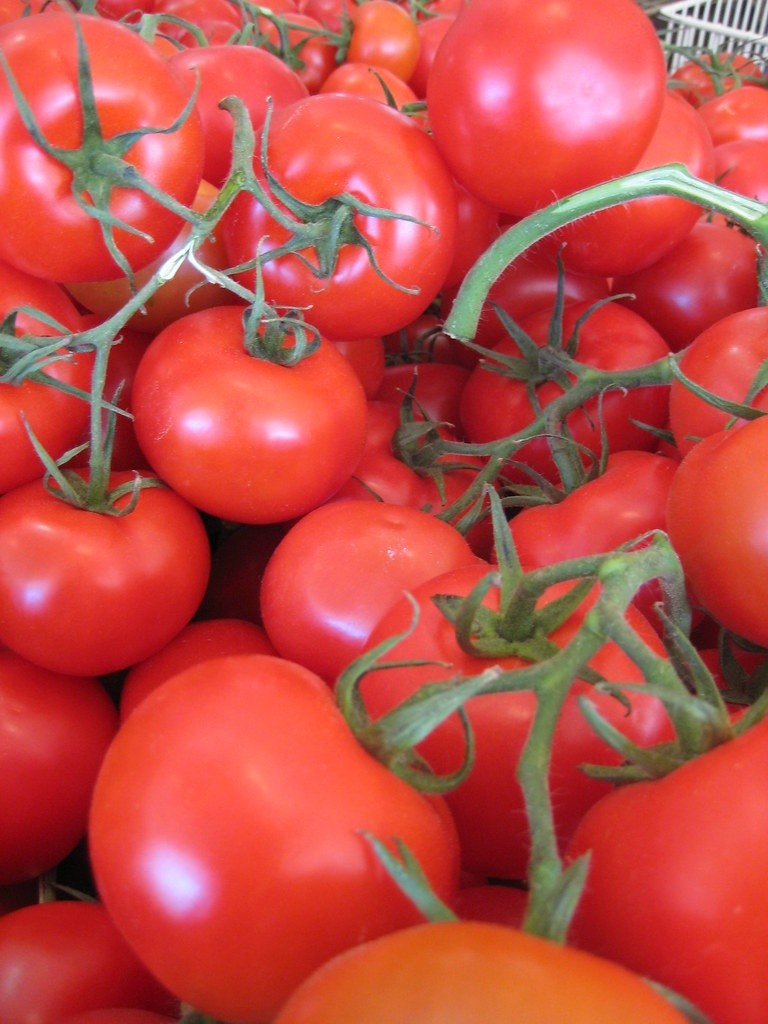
[0,0,768,1023]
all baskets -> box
[656,0,768,77]
